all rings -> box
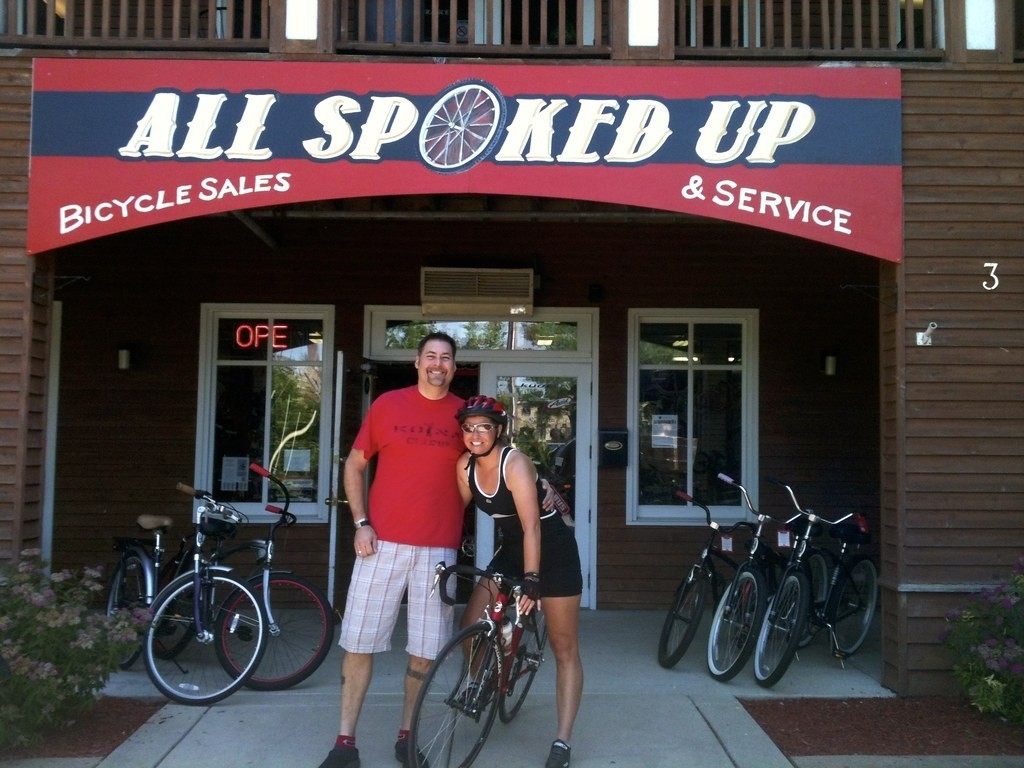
[357,551,361,554]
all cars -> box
[537,432,676,494]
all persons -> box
[455,395,584,768]
[550,422,570,441]
[314,332,554,768]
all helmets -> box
[454,396,507,434]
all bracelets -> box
[355,518,368,528]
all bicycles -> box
[406,563,546,768]
[148,464,337,691]
[108,481,266,707]
[658,474,881,688]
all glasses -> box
[461,423,499,433]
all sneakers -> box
[546,740,570,768]
[395,741,428,768]
[318,745,360,768]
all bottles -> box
[501,615,513,656]
